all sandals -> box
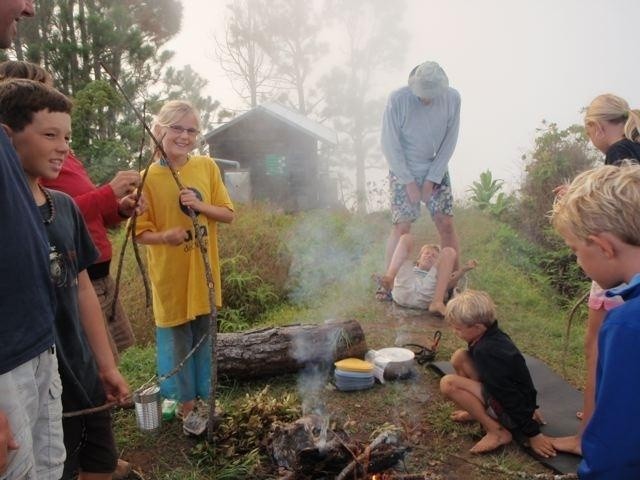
[374,282,394,303]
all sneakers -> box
[181,397,225,438]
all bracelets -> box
[160,231,167,245]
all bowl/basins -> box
[376,347,415,379]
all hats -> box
[406,60,451,102]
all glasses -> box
[162,124,200,136]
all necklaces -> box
[39,181,55,226]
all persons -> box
[373,59,463,302]
[439,291,558,457]
[546,94,640,456]
[125,101,236,438]
[545,157,639,478]
[2,0,69,480]
[370,233,478,319]
